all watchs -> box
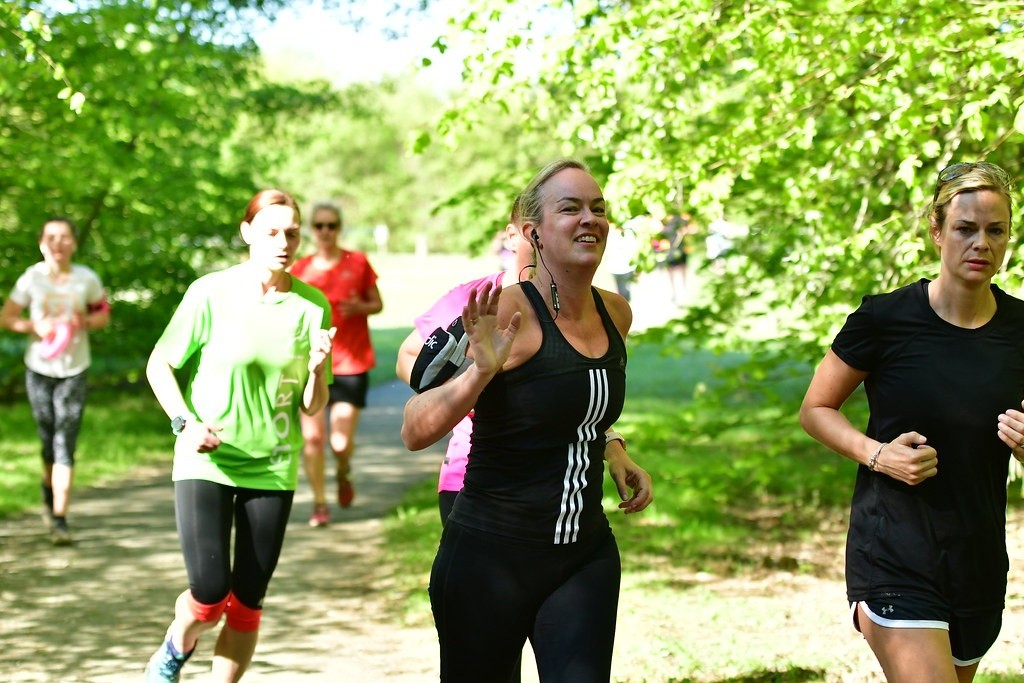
[606,432,627,450]
[171,412,197,435]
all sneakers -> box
[308,504,331,528]
[49,524,72,547]
[338,478,354,507]
[40,497,53,526]
[142,626,198,683]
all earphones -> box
[533,233,540,248]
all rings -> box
[1019,443,1024,448]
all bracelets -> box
[870,442,887,471]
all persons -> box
[146,189,337,683]
[289,203,384,530]
[394,190,537,536]
[400,160,654,683]
[0,218,110,545]
[608,221,632,303]
[660,212,691,292]
[799,160,1024,683]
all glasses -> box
[314,222,338,230]
[934,163,1012,201]
